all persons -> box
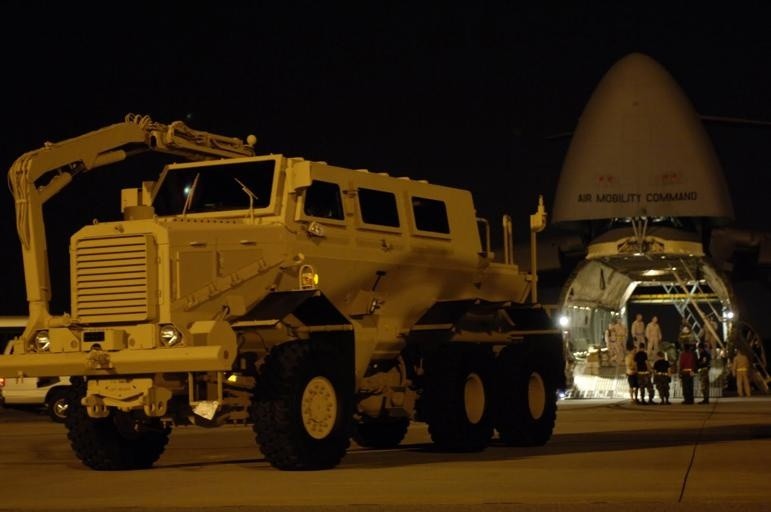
[731,350,751,395]
[604,314,719,404]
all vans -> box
[0,315,78,421]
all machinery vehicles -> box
[0,109,569,470]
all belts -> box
[656,372,667,375]
[738,368,747,371]
[638,371,649,374]
[683,368,692,372]
